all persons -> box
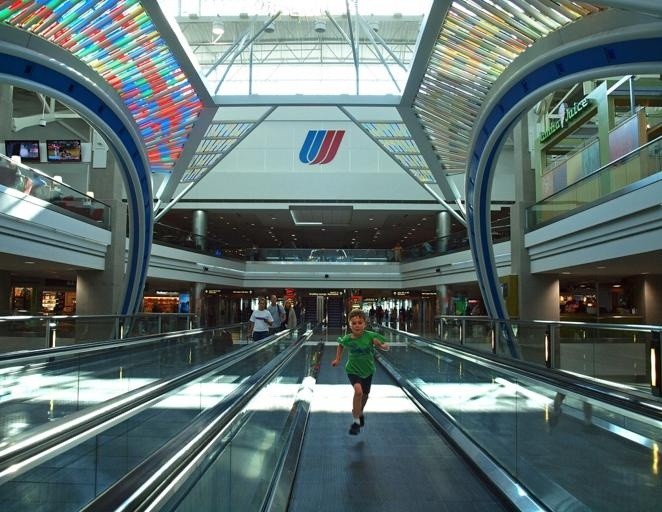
[369,305,415,323]
[249,297,275,371]
[30,166,47,189]
[282,296,298,350]
[208,231,299,261]
[330,308,392,437]
[391,240,404,262]
[8,141,80,160]
[7,154,24,188]
[267,293,286,355]
[374,321,414,342]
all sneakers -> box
[360,415,364,426]
[349,423,360,435]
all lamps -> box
[313,18,327,31]
[368,17,379,32]
[264,15,275,32]
[38,93,47,127]
[212,12,226,34]
